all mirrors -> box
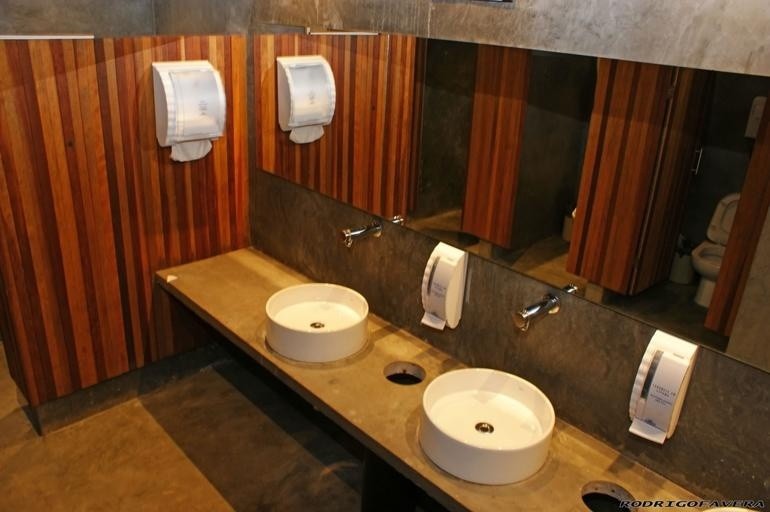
[253,25,770,375]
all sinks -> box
[264,282,369,364]
[419,367,556,485]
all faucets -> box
[562,282,579,293]
[341,219,383,249]
[512,291,561,335]
[389,213,410,228]
